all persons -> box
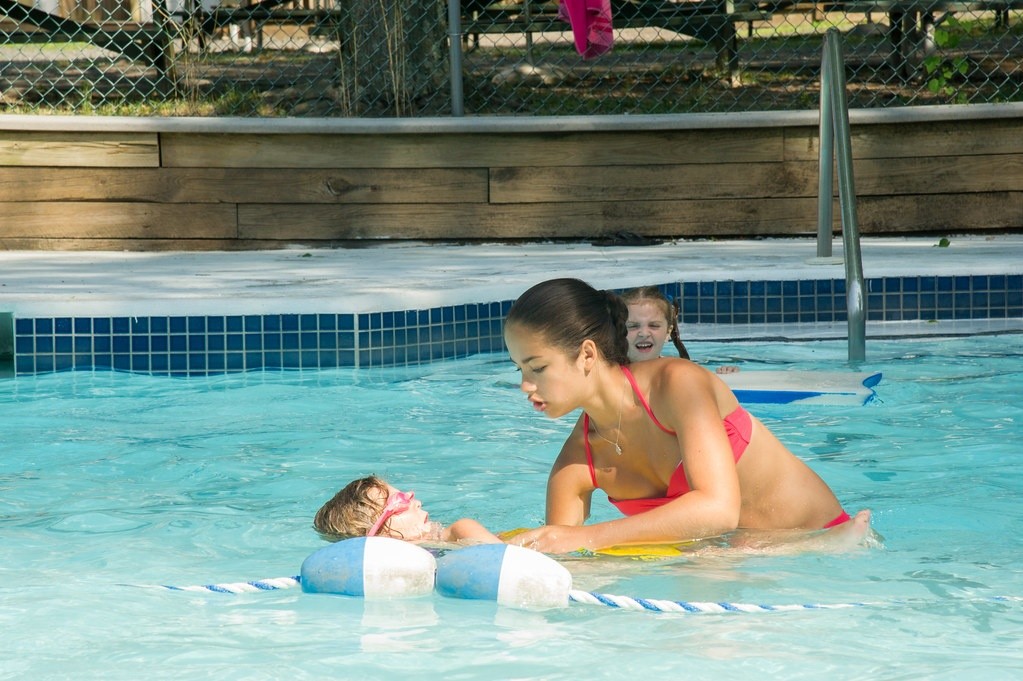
[503,278,885,554]
[313,474,872,580]
[621,286,741,375]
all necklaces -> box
[588,374,625,456]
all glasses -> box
[367,491,414,537]
